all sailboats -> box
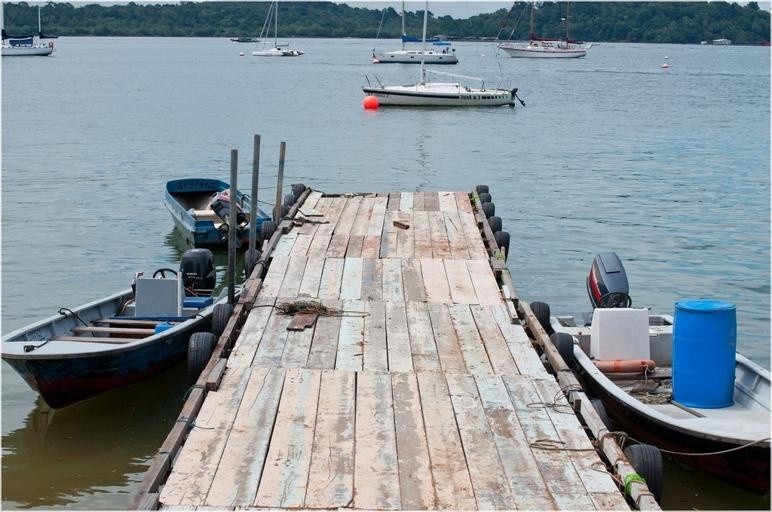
[32,3,58,39]
[248,0,304,56]
[361,0,590,107]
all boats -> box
[158,175,277,252]
[0,248,251,412]
[548,248,770,498]
[232,34,261,43]
[0,27,53,55]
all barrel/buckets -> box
[671,298,737,410]
[363,95,379,109]
